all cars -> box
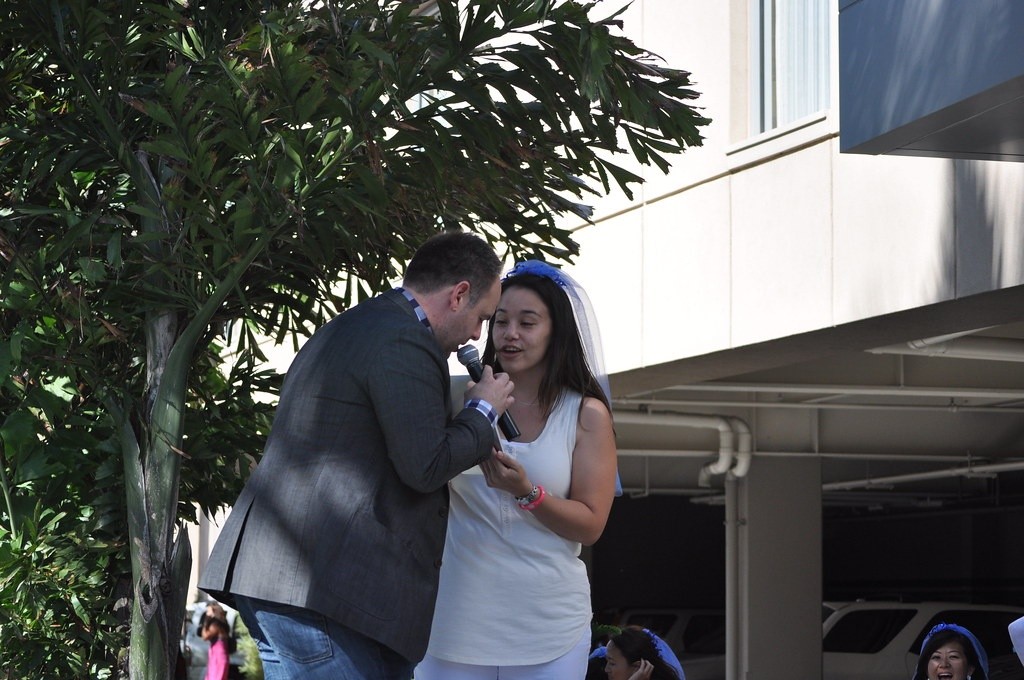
[180,600,249,680]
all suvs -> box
[822,599,1024,680]
[619,605,726,680]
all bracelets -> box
[518,485,545,511]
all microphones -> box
[457,345,521,443]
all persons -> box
[197,232,516,680]
[912,624,989,680]
[586,625,686,680]
[202,601,230,680]
[414,261,623,680]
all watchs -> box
[512,485,538,505]
